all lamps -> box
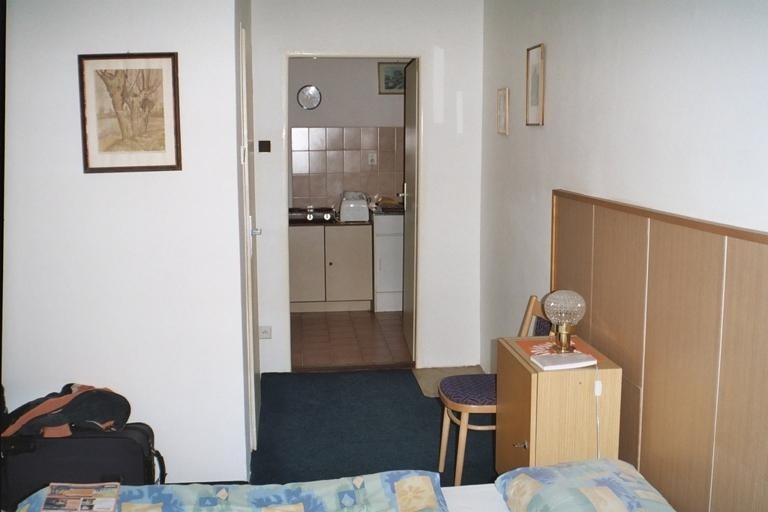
[544,289,588,353]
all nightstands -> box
[495,335,623,475]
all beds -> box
[17,188,768,512]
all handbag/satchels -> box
[1,384,132,439]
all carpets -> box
[164,367,500,487]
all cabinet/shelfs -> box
[288,225,373,313]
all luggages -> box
[0,422,166,511]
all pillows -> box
[494,459,678,512]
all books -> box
[529,349,598,372]
[42,481,120,512]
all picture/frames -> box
[497,88,509,136]
[378,61,408,94]
[78,52,182,174]
[526,44,544,126]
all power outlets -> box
[368,152,377,166]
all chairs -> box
[438,295,558,486]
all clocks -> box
[297,85,321,110]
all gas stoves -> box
[288,205,336,224]
